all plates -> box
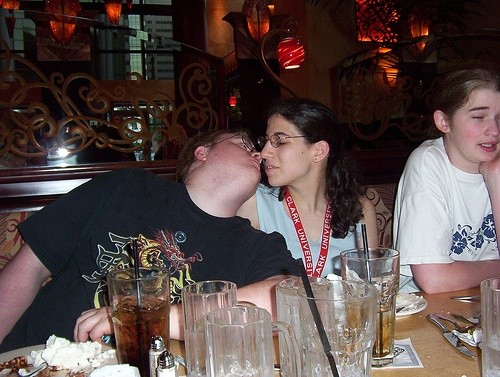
[394,292,427,320]
[0,343,118,377]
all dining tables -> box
[171,287,483,377]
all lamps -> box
[276,36,306,70]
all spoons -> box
[435,313,476,335]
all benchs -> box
[1,156,404,286]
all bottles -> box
[156,350,175,377]
[149,335,168,377]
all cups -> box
[341,248,400,368]
[204,306,303,377]
[275,277,308,377]
[181,280,238,377]
[480,277,500,377]
[297,280,377,377]
[107,267,170,377]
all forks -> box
[450,313,482,324]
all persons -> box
[237,98,378,279]
[0,132,302,353]
[392,68,500,295]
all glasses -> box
[257,134,316,148]
[192,132,256,157]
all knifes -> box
[426,313,477,358]
[425,313,476,360]
